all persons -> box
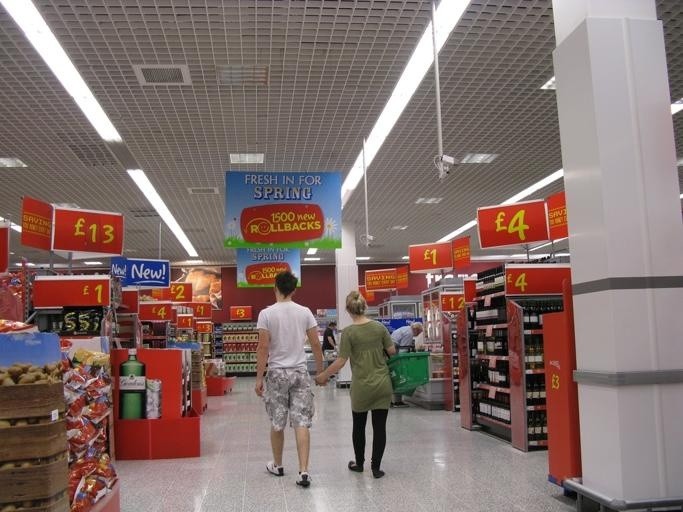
[383,322,424,408]
[313,291,397,478]
[322,322,338,378]
[254,271,323,486]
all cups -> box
[143,379,162,419]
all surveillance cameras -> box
[434,154,455,174]
[367,235,373,246]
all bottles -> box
[469,263,511,423]
[512,300,563,444]
[118,349,146,419]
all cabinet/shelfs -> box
[1,323,121,511]
[304,319,334,374]
[21,288,222,460]
[378,261,569,453]
[222,323,260,376]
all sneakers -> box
[348,461,363,472]
[296,472,310,487]
[394,401,409,407]
[267,461,284,476]
[373,471,385,478]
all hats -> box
[412,322,423,330]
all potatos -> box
[0,362,68,512]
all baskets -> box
[384,346,430,394]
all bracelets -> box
[323,370,330,377]
